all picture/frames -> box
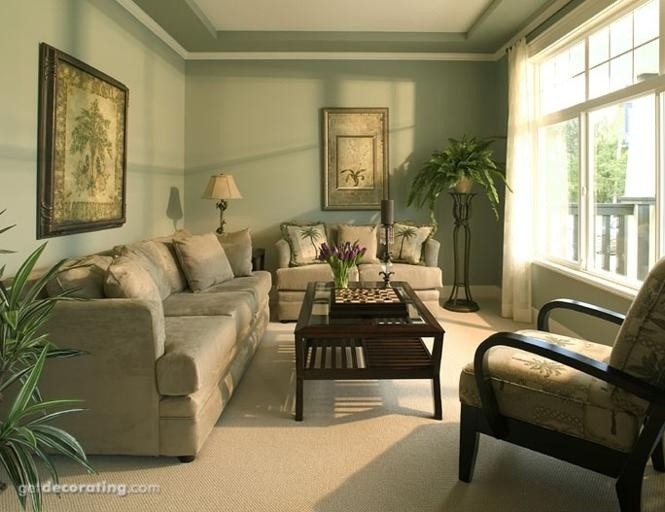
[320,106,390,212]
[35,40,130,243]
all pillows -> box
[383,220,440,267]
[43,224,255,314]
[336,224,382,265]
[279,219,331,268]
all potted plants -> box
[404,133,514,224]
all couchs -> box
[1,229,273,464]
[274,223,444,325]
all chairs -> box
[456,253,665,512]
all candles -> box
[380,199,397,228]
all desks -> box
[252,248,266,271]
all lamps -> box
[200,172,243,234]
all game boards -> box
[330,288,409,319]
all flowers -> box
[317,238,368,288]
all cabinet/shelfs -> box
[442,192,481,313]
